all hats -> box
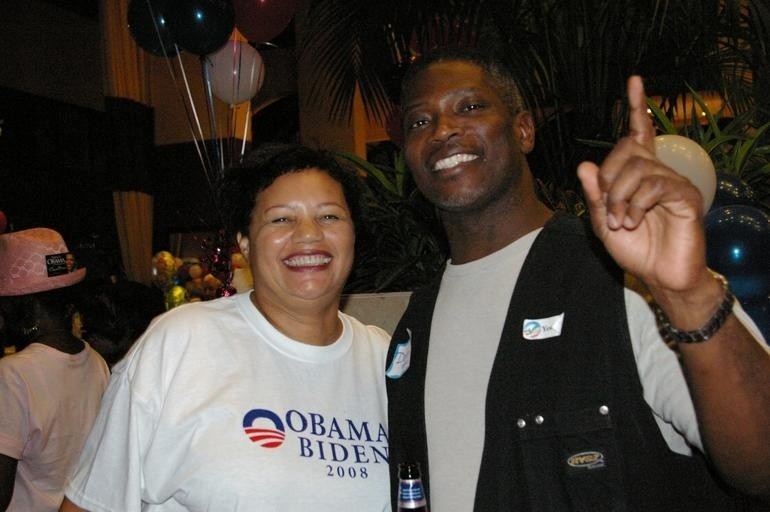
[0,226,88,297]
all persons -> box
[64,250,77,274]
[386,38,770,511]
[57,144,392,511]
[1,227,112,510]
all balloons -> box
[711,170,769,215]
[652,132,717,220]
[703,203,770,305]
[125,0,185,58]
[232,2,297,39]
[169,0,237,57]
[205,37,268,108]
[148,245,239,315]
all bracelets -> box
[653,267,732,346]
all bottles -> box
[397,462,428,512]
